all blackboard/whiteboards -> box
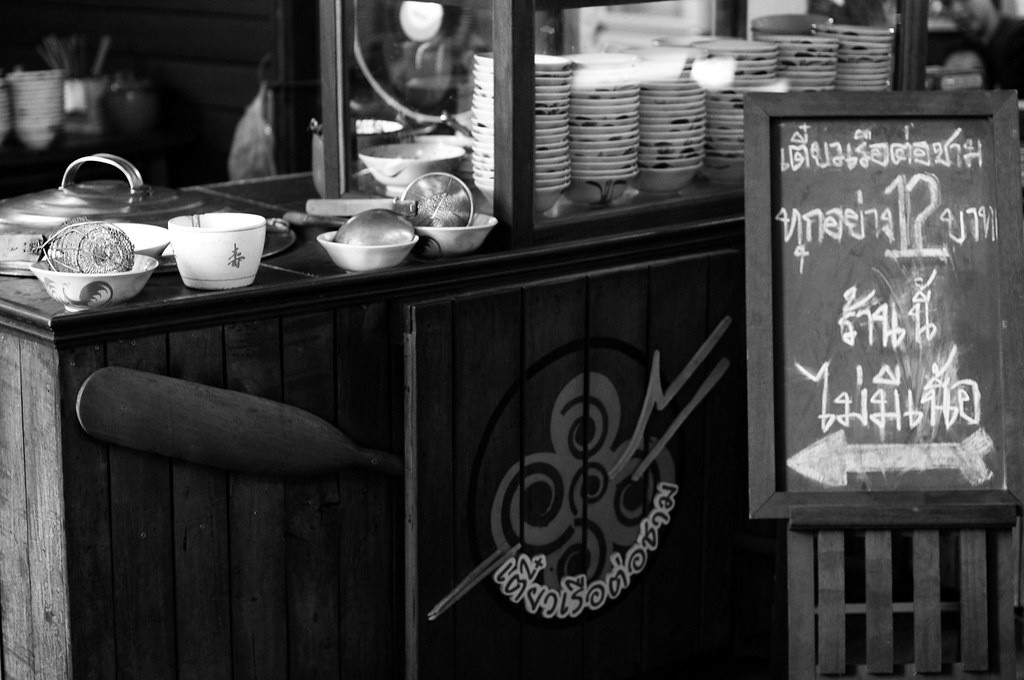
[738,89,1023,526]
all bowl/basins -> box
[29,255,159,313]
[0,69,67,152]
[409,212,498,263]
[311,13,895,219]
[317,231,420,275]
[105,222,172,258]
[168,212,266,291]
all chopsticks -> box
[35,31,112,78]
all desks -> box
[0,128,200,198]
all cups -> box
[58,74,108,138]
